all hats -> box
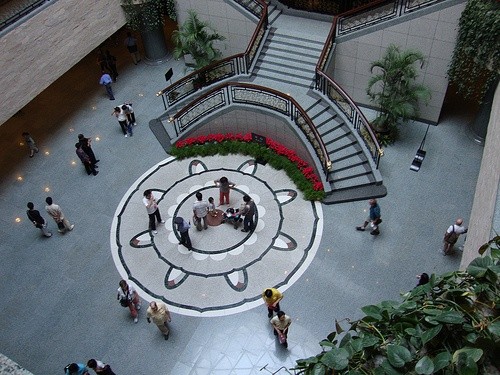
[175,217,183,223]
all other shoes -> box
[188,246,191,251]
[214,215,217,217]
[58,228,64,232]
[215,211,218,214]
[165,334,168,340]
[30,155,34,158]
[110,98,115,100]
[226,203,229,207]
[242,228,248,232]
[440,250,445,256]
[125,122,137,137]
[36,149,39,153]
[135,303,141,310]
[69,225,74,230]
[217,204,223,207]
[154,230,158,234]
[283,342,287,348]
[88,160,100,176]
[161,220,165,224]
[134,314,139,323]
[179,241,185,244]
[356,226,366,231]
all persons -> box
[214,177,236,207]
[174,217,192,251]
[125,34,141,64]
[26,201,52,238]
[356,198,381,235]
[45,197,74,233]
[99,71,115,100]
[145,301,171,340]
[114,104,137,138]
[23,132,39,157]
[75,134,100,176]
[64,359,114,375]
[263,288,283,318]
[229,194,256,232]
[143,190,165,233]
[415,273,430,287]
[440,218,465,256]
[95,50,119,82]
[193,192,218,231]
[270,311,292,348]
[117,280,141,323]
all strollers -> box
[221,208,243,230]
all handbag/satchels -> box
[279,335,286,344]
[119,299,130,307]
[445,224,457,244]
[374,219,382,226]
[269,305,277,311]
